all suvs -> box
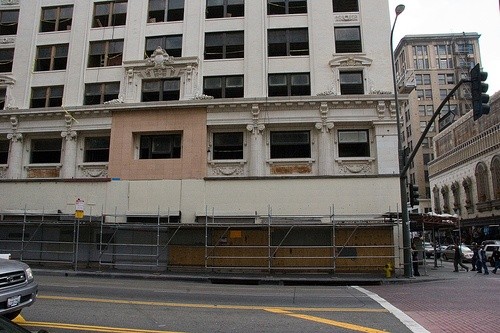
[484,245,500,261]
[422,242,434,256]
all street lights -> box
[391,5,413,277]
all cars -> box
[443,244,474,262]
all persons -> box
[412,242,421,277]
[450,240,500,275]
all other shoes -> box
[467,267,468,272]
[491,271,496,274]
[484,272,489,275]
[471,269,476,271]
[478,271,482,273]
[453,270,458,272]
